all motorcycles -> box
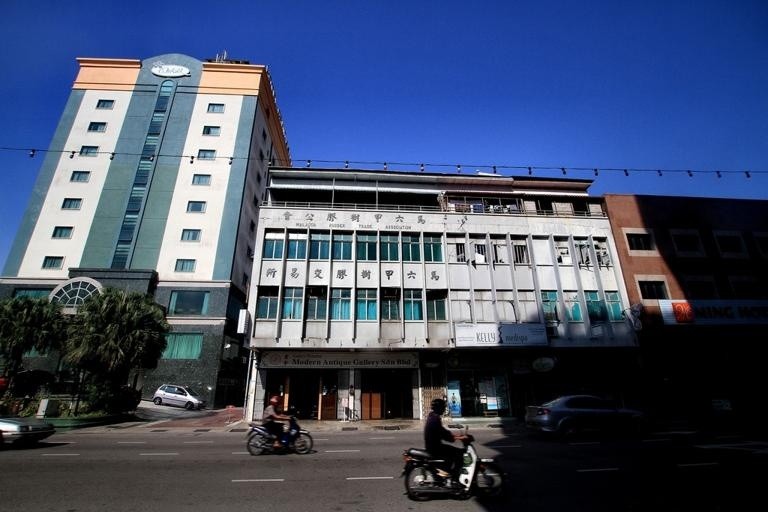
[399,421,503,501]
[242,414,314,456]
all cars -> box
[524,394,645,443]
[0,405,57,448]
[152,383,207,411]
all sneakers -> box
[451,481,466,490]
[272,440,283,447]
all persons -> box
[424,399,470,488]
[262,395,291,449]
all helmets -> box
[270,395,284,406]
[430,399,447,411]
